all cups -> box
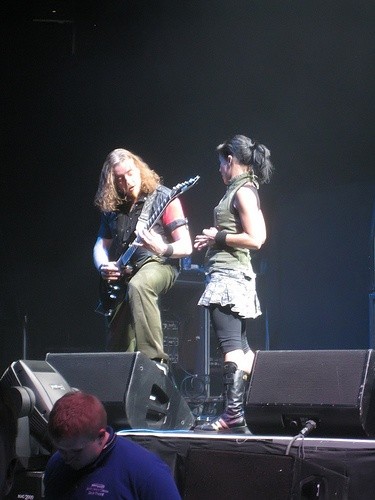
[179,256,191,270]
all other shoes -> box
[155,361,169,375]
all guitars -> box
[101,174,202,327]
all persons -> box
[192,134,276,434]
[43,390,181,500]
[94,148,192,401]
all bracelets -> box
[99,264,102,270]
[215,231,227,247]
[163,244,173,257]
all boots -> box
[194,361,249,434]
